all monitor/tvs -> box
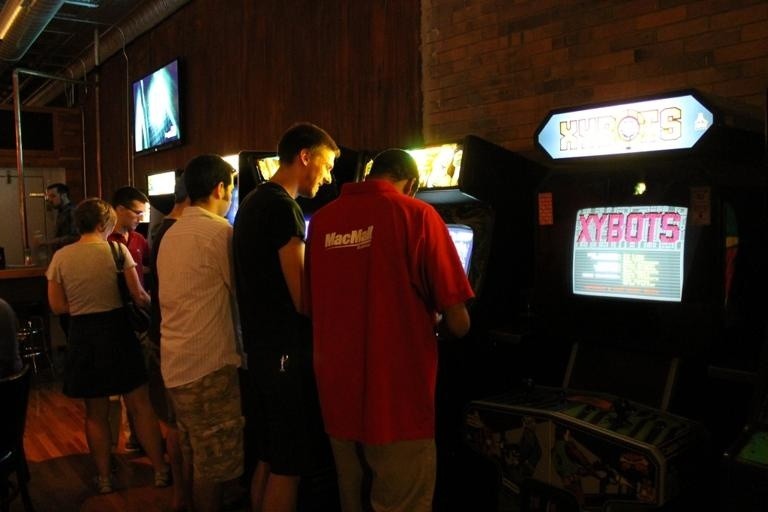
[444,221,474,279]
[570,203,690,305]
[130,56,184,156]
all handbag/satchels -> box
[130,306,160,377]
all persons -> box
[108,187,151,453]
[46,198,168,493]
[231,122,341,512]
[0,298,20,374]
[300,149,476,512]
[151,172,194,512]
[44,182,81,252]
[157,155,244,512]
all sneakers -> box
[152,464,171,487]
[94,474,111,494]
[124,443,141,452]
